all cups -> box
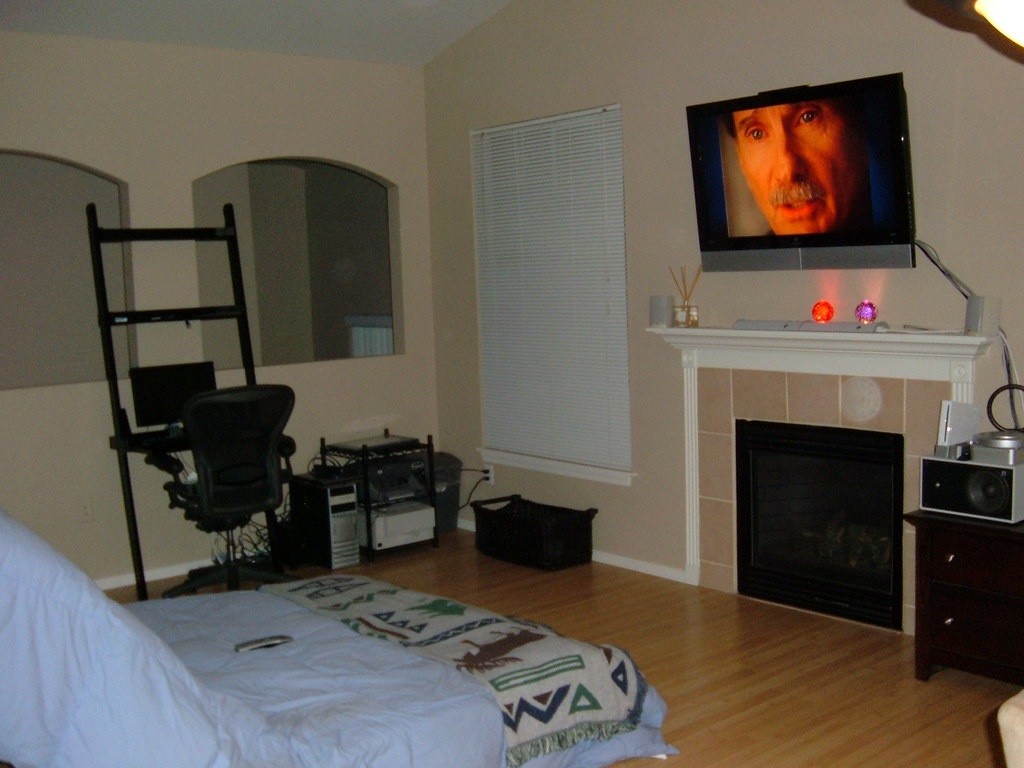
[649,294,676,328]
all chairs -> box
[139,384,296,596]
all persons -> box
[724,98,862,234]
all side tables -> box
[904,510,1024,685]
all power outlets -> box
[483,464,494,486]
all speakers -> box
[963,295,1001,338]
[649,294,675,327]
[919,455,1024,524]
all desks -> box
[86,199,304,601]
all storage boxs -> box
[473,494,600,572]
[354,502,436,550]
[415,450,463,532]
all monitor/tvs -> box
[684,72,916,272]
[128,361,216,432]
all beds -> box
[0,507,683,768]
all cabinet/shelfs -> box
[320,428,441,557]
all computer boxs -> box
[290,473,361,569]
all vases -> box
[673,299,699,329]
